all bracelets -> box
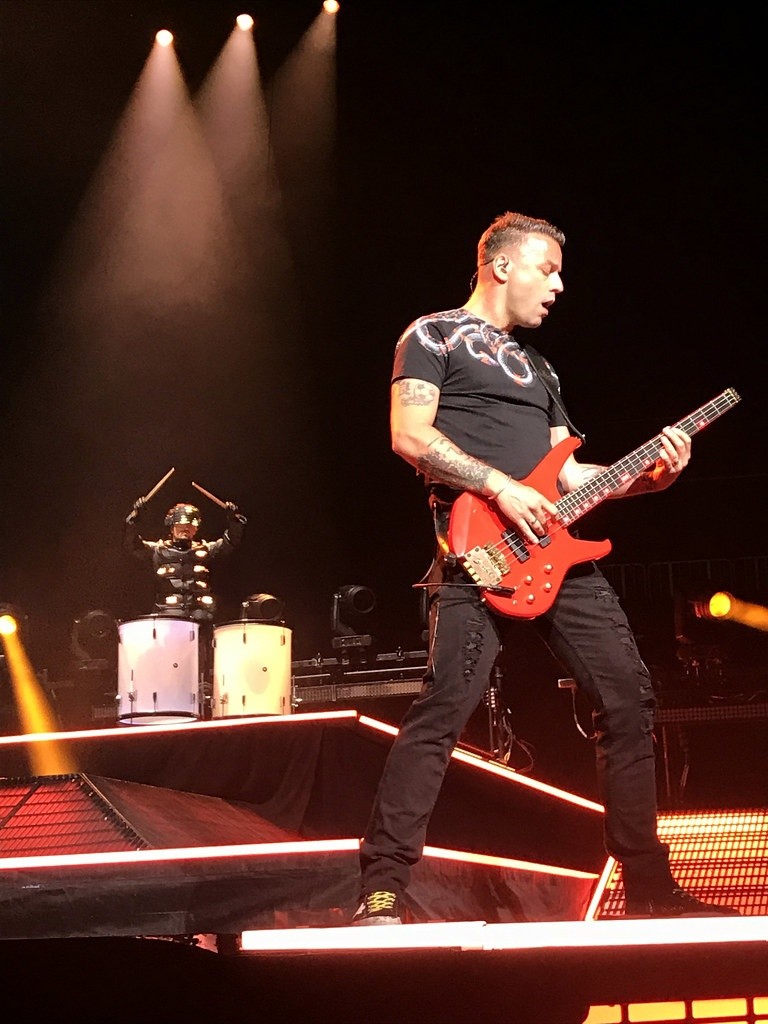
[488,474,512,500]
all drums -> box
[112,613,207,727]
[210,618,295,720]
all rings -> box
[530,516,537,526]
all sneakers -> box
[351,886,404,927]
[624,882,738,916]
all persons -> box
[348,211,742,928]
[121,496,245,722]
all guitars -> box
[447,387,745,621]
